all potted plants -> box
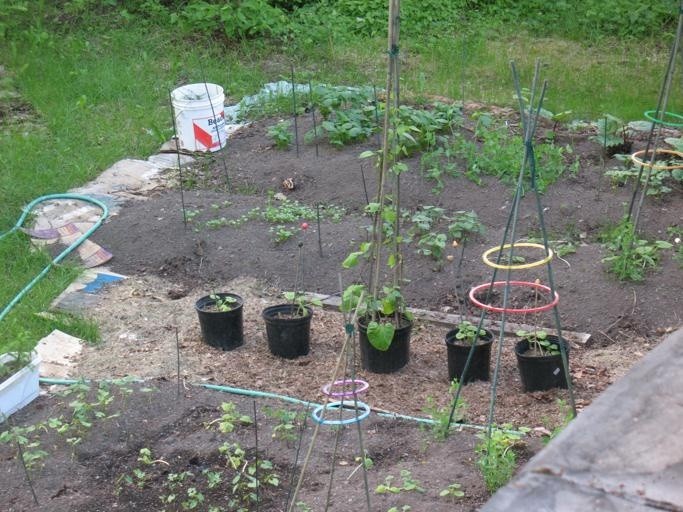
[0,330,42,424]
[515,330,571,392]
[196,294,244,349]
[261,290,323,360]
[340,89,415,375]
[444,321,496,383]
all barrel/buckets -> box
[171,82,227,153]
